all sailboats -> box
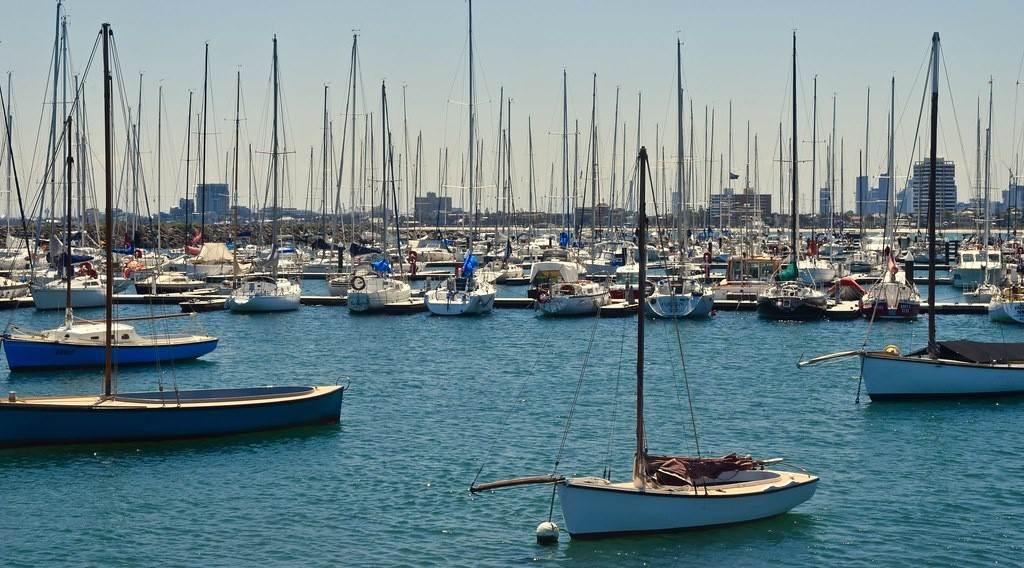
[0,0,1024,374]
[1,23,345,450]
[470,145,825,544]
[795,32,1023,404]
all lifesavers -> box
[643,280,656,298]
[123,267,132,279]
[351,275,367,291]
[535,290,550,304]
